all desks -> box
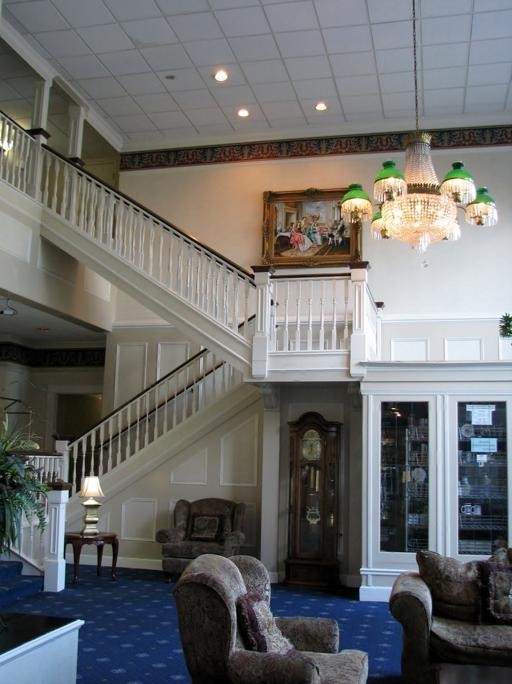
[1,612,85,684]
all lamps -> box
[79,476,106,538]
[339,1,499,254]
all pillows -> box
[236,592,294,656]
[484,549,512,624]
[416,549,490,621]
[189,511,223,542]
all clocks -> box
[283,411,345,588]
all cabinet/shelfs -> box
[359,381,512,602]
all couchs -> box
[389,550,512,684]
[174,554,369,684]
[155,498,247,583]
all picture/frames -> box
[250,187,369,272]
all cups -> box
[460,502,471,514]
[470,503,481,515]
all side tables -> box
[63,532,119,583]
